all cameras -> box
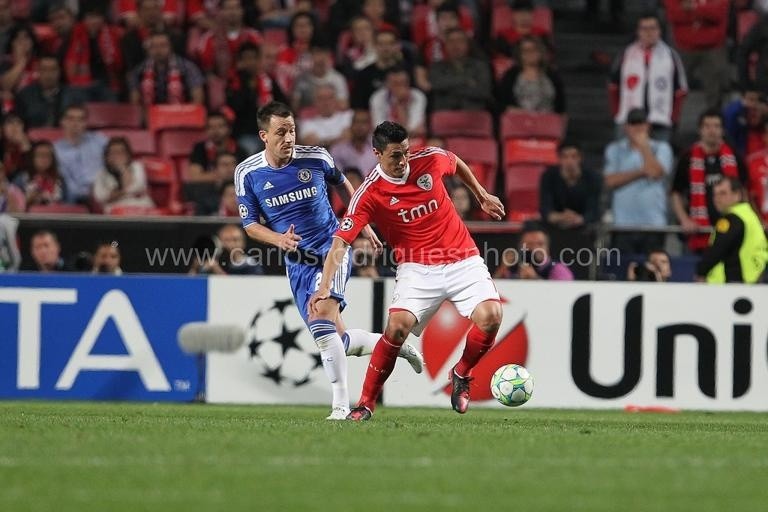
[634,258,653,276]
[520,247,534,266]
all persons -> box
[0,0,768,286]
[303,120,509,423]
[229,101,424,424]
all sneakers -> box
[326,406,374,422]
[447,364,474,414]
[402,343,424,374]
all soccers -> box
[245,299,321,387]
[490,364,534,406]
